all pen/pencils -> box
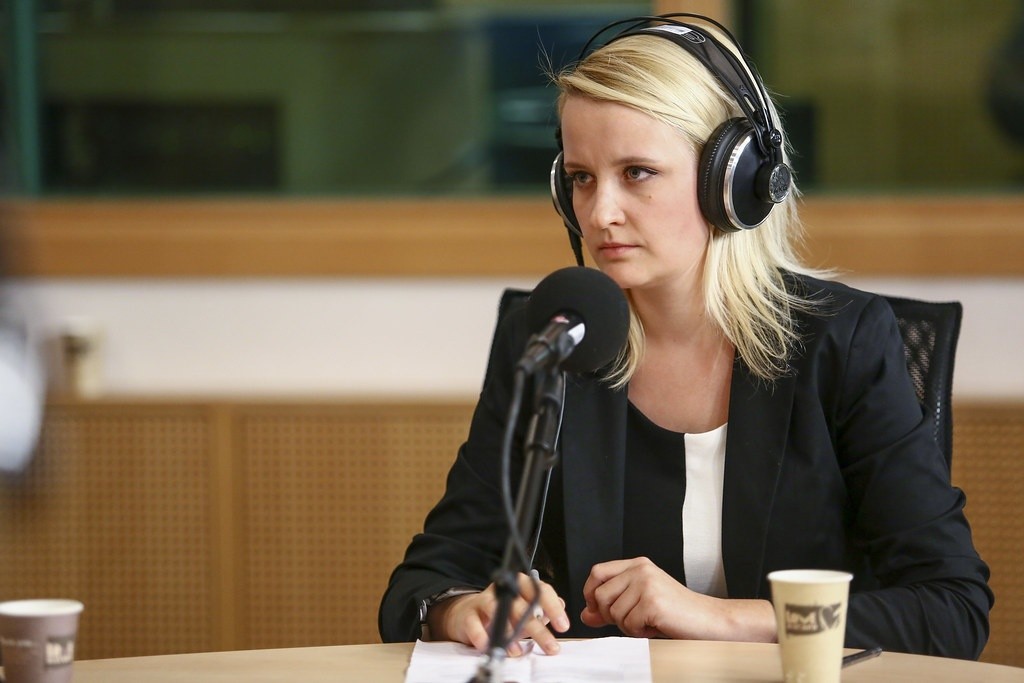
[529,568,544,622]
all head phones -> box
[548,14,792,232]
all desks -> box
[69,636,1024,683]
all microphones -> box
[515,265,629,387]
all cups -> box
[767,569,854,683]
[0,599,85,683]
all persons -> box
[378,23,994,660]
